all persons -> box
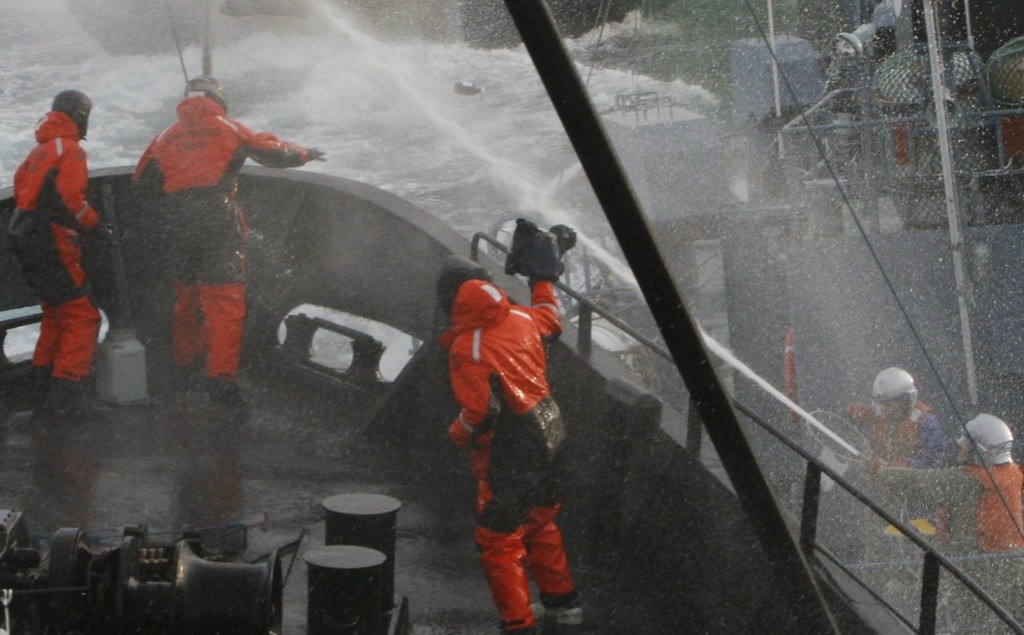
[436,256,584,635]
[10,89,112,418]
[839,369,1024,554]
[126,73,327,406]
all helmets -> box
[184,76,227,112]
[958,411,1014,464]
[872,367,918,411]
[53,90,92,134]
[437,261,483,310]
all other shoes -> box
[34,371,88,396]
[212,382,250,407]
[530,601,581,624]
[174,371,193,385]
